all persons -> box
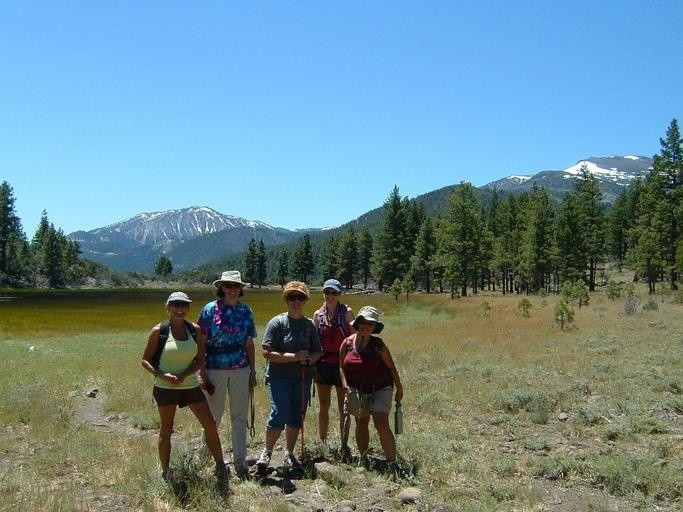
[140,291,229,481]
[309,278,354,462]
[195,270,258,482]
[258,280,324,469]
[336,306,404,469]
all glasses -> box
[168,302,189,308]
[324,289,342,296]
[219,283,243,289]
[285,294,306,302]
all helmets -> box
[323,278,343,292]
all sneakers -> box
[254,451,272,467]
[283,453,300,468]
[161,468,173,483]
[214,464,229,480]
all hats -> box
[281,280,311,302]
[165,291,193,307]
[213,270,247,288]
[348,305,385,335]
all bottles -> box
[395,399,404,434]
